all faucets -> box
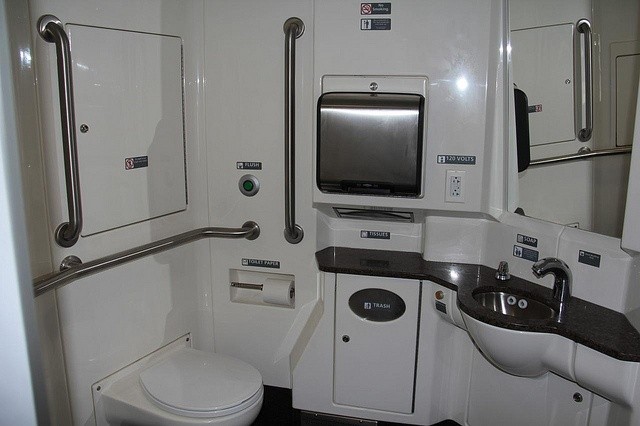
[532,257,573,302]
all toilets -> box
[103,346,263,426]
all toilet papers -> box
[262,277,293,306]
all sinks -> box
[472,286,557,320]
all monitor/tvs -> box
[316,92,425,197]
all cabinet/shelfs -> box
[64,22,189,239]
[509,23,577,147]
[334,271,422,414]
[466,346,594,426]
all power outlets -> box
[446,170,466,203]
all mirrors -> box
[508,2,640,238]
